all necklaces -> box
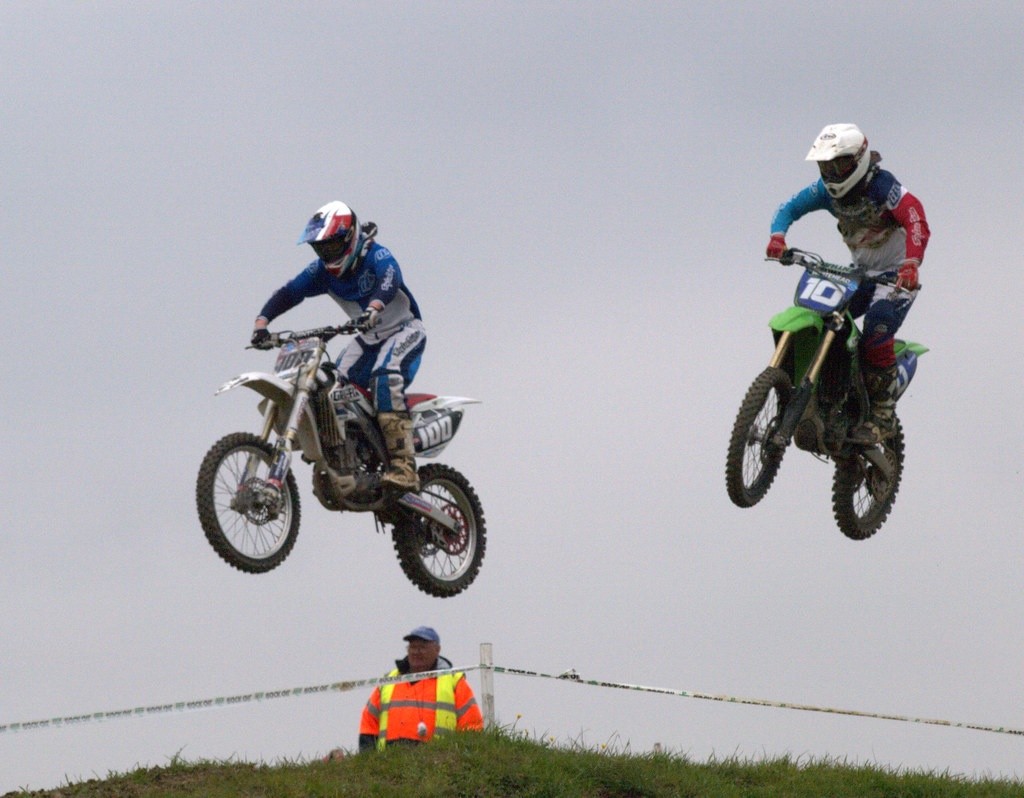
[410,680,427,735]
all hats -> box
[403,626,439,642]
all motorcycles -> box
[197,310,489,597]
[723,240,930,542]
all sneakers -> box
[382,458,420,493]
[845,410,898,446]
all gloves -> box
[251,328,274,350]
[895,264,918,292]
[346,305,383,334]
[766,235,788,258]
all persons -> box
[358,627,484,758]
[764,122,930,445]
[251,200,426,492]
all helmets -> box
[295,200,362,280]
[805,123,870,198]
